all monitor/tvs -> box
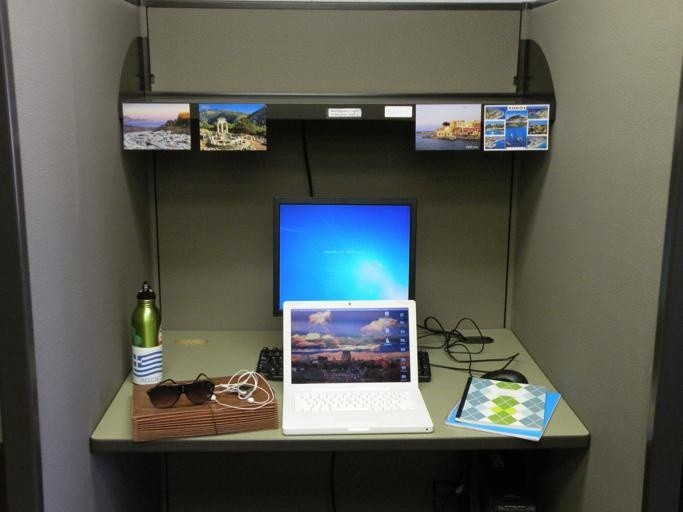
[273,196,417,317]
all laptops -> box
[281,299,434,436]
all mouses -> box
[481,369,529,384]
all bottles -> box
[132,283,164,387]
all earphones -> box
[205,394,216,401]
[246,397,254,403]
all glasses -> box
[146,373,214,409]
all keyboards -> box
[256,346,431,381]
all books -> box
[442,389,563,444]
[455,376,548,432]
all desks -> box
[90,329,590,454]
